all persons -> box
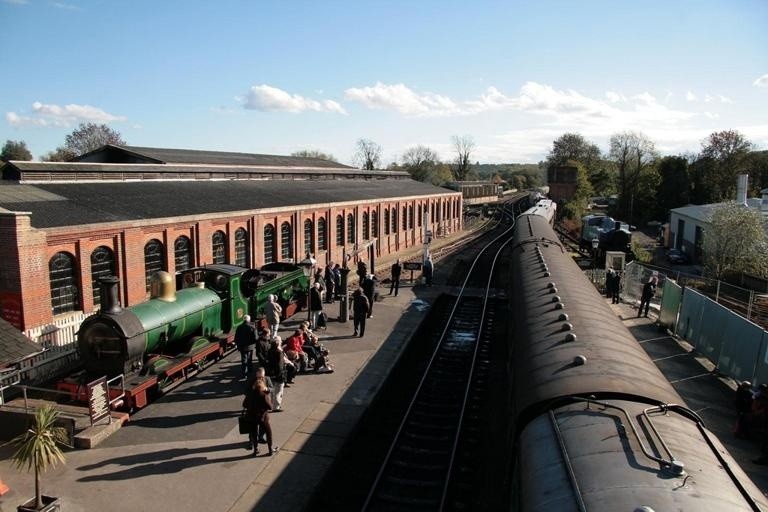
[637,275,658,318]
[388,258,401,297]
[233,292,334,459]
[423,253,435,287]
[314,259,375,340]
[309,282,325,331]
[606,265,620,304]
[730,380,767,466]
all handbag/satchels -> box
[238,409,254,434]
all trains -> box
[508,185,768,512]
[582,213,633,262]
[55,258,311,413]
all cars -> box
[664,246,689,266]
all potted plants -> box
[1,403,72,511]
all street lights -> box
[299,250,318,329]
